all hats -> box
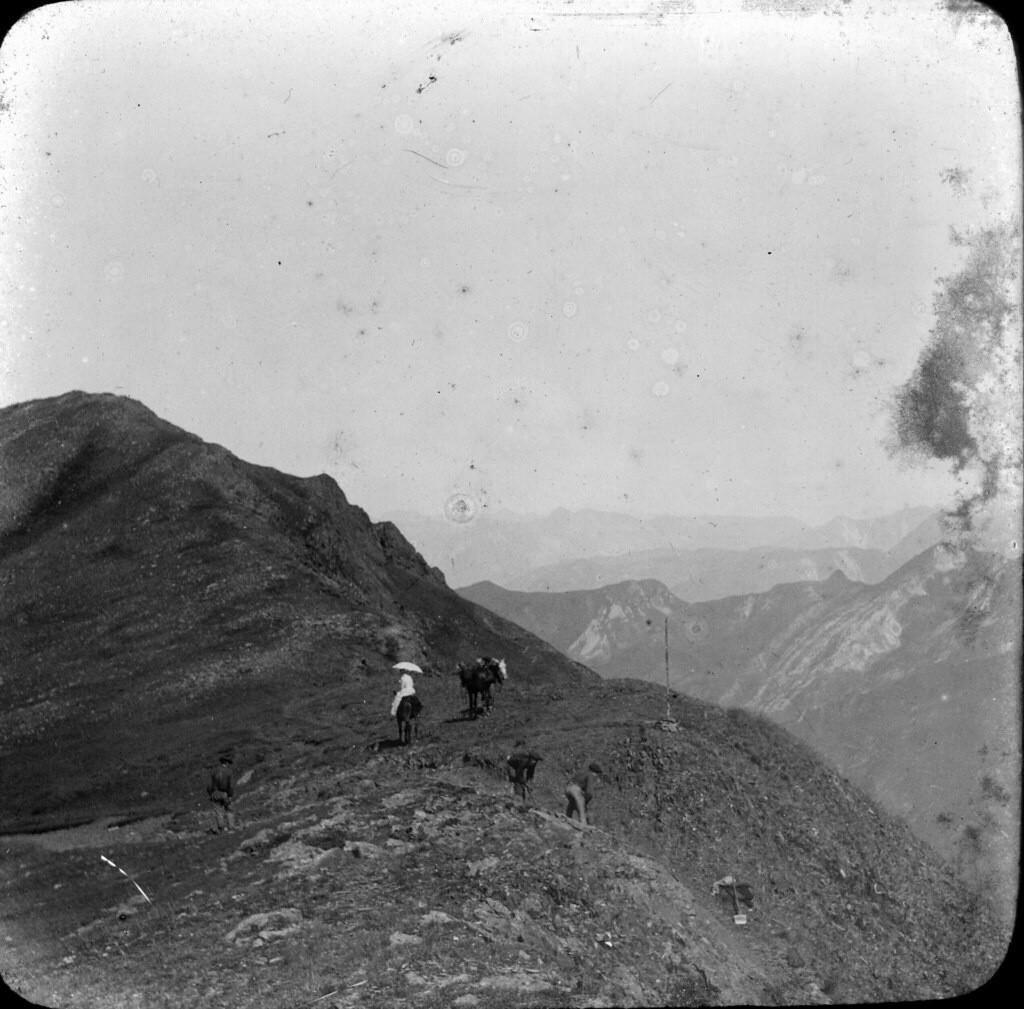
[529,751,542,760]
[590,764,603,773]
[218,755,233,764]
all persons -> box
[506,751,544,801]
[207,756,238,834]
[565,764,603,825]
[391,669,415,716]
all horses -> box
[394,689,423,747]
[458,656,509,718]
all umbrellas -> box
[392,662,423,674]
